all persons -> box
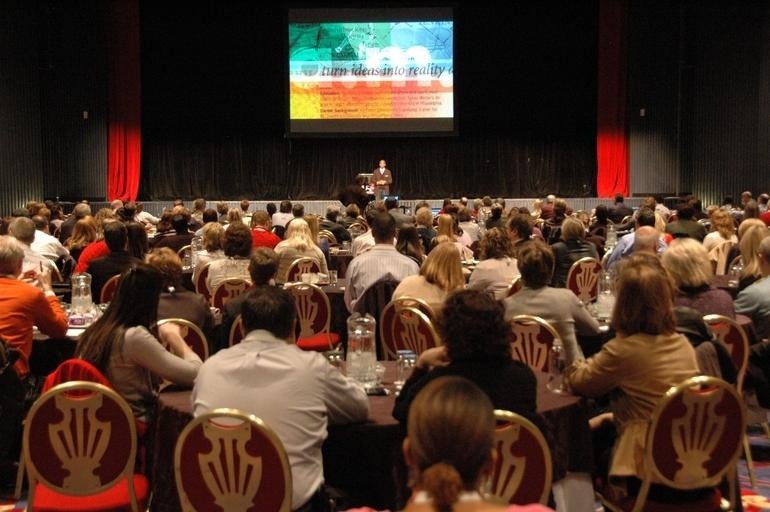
[1,189,770,418]
[185,284,368,512]
[561,261,721,507]
[69,263,209,471]
[357,174,376,202]
[497,239,613,476]
[336,175,376,218]
[343,375,553,511]
[392,286,558,512]
[369,160,395,203]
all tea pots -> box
[69,274,93,315]
[605,224,616,247]
[192,233,203,270]
[596,271,617,319]
[346,312,378,387]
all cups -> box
[301,269,338,287]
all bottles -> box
[58,302,111,327]
[323,350,345,367]
[393,350,417,387]
[546,338,567,394]
